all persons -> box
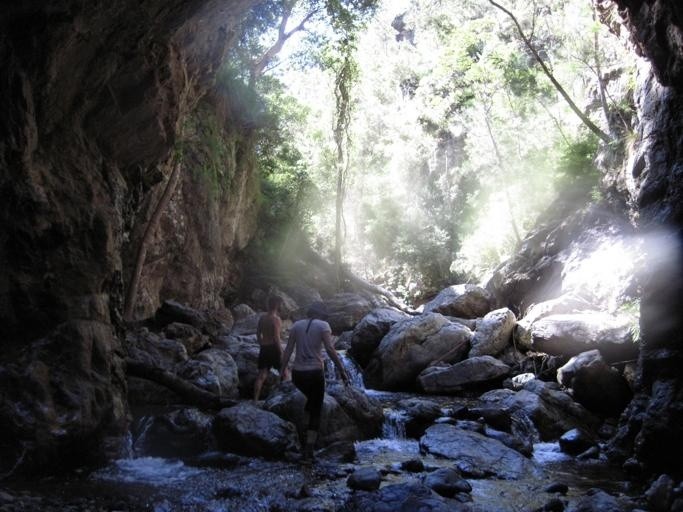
[279,301,348,462]
[254,297,288,402]
